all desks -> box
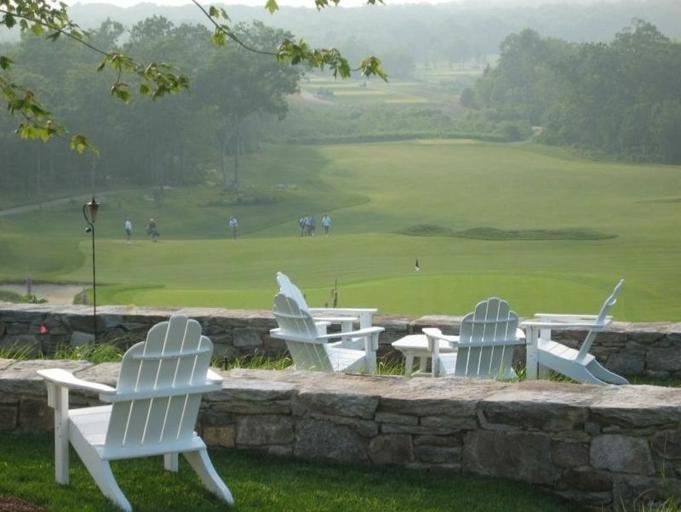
[390,333,462,376]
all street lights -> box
[83,198,99,314]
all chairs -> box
[34,312,236,511]
[275,272,378,352]
[520,275,629,385]
[269,292,385,374]
[421,295,525,381]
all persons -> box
[297,213,314,237]
[145,216,160,242]
[321,215,332,233]
[229,216,240,239]
[124,217,133,240]
